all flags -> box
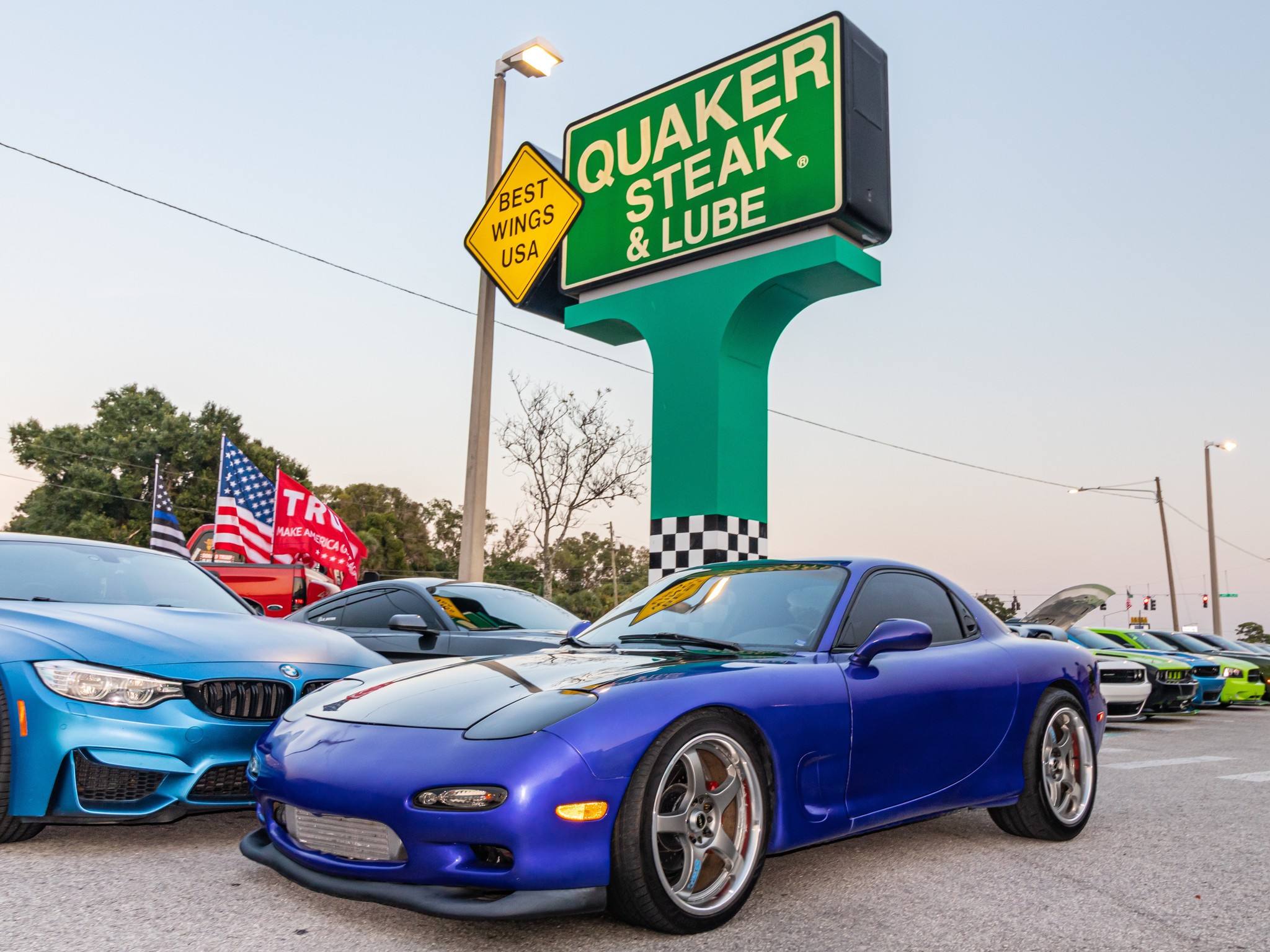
[149,464,191,560]
[269,468,368,593]
[212,431,318,564]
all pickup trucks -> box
[185,523,344,618]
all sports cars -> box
[236,556,1105,938]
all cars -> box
[0,532,395,844]
[1003,582,1270,723]
[280,576,582,665]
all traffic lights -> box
[1143,598,1149,610]
[1150,599,1156,610]
[1017,602,1021,610]
[1203,594,1208,608]
[1100,603,1107,610]
[1012,602,1015,611]
[1013,596,1018,602]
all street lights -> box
[1067,477,1179,631]
[1203,439,1238,637]
[458,36,568,581]
